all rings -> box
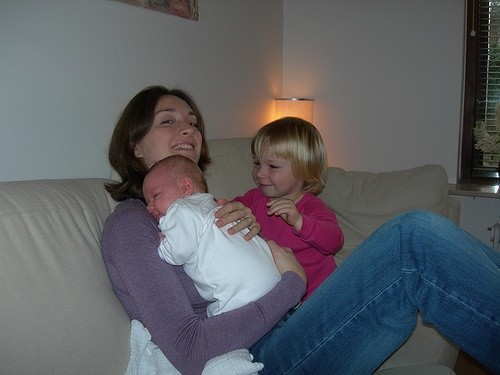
[247,217,252,226]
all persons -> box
[230,116,345,304]
[142,154,282,318]
[101,84,500,375]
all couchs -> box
[0,137,460,375]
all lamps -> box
[273,97,315,125]
